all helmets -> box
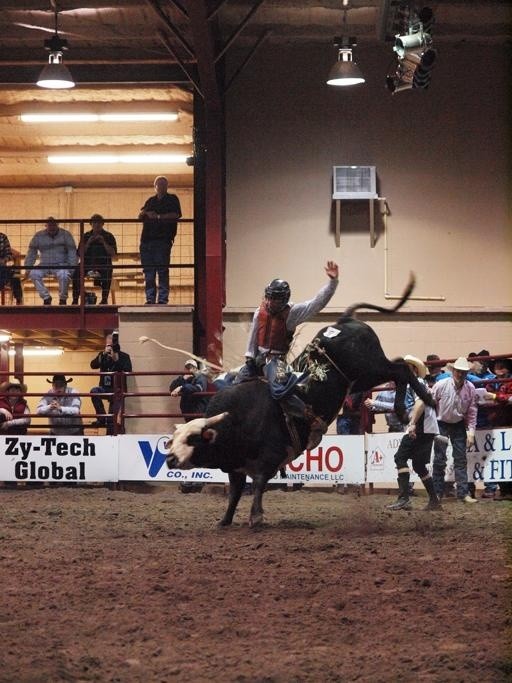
[264,279,291,314]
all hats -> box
[469,349,490,367]
[0,378,27,399]
[184,359,197,368]
[445,357,470,373]
[46,375,73,385]
[485,359,512,375]
[422,355,446,367]
[403,354,426,380]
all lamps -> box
[33,0,77,93]
[324,2,368,89]
[385,9,436,96]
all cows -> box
[164,270,438,529]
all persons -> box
[0,378,31,435]
[25,216,79,306]
[71,215,118,305]
[36,374,84,435]
[169,359,208,425]
[139,176,182,305]
[337,349,512,511]
[232,260,339,451]
[90,334,132,435]
[0,231,23,305]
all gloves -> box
[246,359,258,376]
[465,430,474,448]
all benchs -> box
[2,251,147,304]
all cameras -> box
[107,333,121,352]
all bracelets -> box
[157,213,161,220]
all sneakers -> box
[99,298,108,304]
[16,296,23,306]
[71,298,78,305]
[43,295,52,305]
[59,298,66,305]
[145,300,166,304]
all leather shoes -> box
[462,495,479,503]
[482,492,512,501]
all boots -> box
[419,476,441,511]
[386,476,410,510]
